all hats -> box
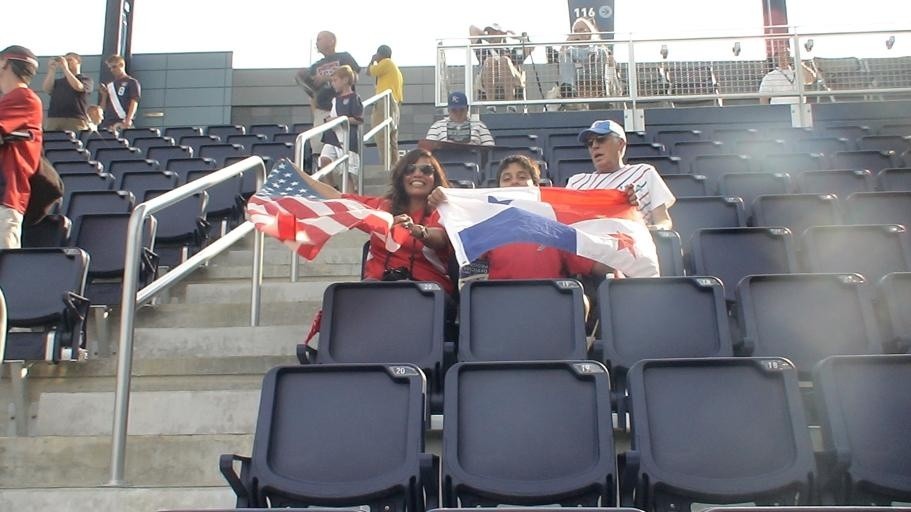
[447,92,469,111]
[578,120,627,144]
[0,45,41,71]
[483,23,509,35]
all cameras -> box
[383,266,411,281]
[55,58,62,62]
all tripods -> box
[515,32,548,115]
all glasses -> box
[585,133,625,146]
[403,163,436,177]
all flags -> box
[244,158,411,261]
[437,187,662,278]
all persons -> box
[466,17,609,112]
[0,45,142,249]
[560,119,677,233]
[295,31,404,190]
[425,92,496,146]
[427,154,662,278]
[286,147,450,348]
[758,47,817,104]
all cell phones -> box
[100,82,106,88]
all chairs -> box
[220,353,906,510]
[293,269,909,359]
[50,173,247,241]
[2,247,94,364]
[36,134,310,146]
[42,144,294,160]
[486,126,908,149]
[50,158,275,193]
[40,124,316,134]
[70,212,160,309]
[64,189,209,266]
[649,228,907,265]
[672,191,909,224]
[436,152,908,172]
[656,172,910,188]
